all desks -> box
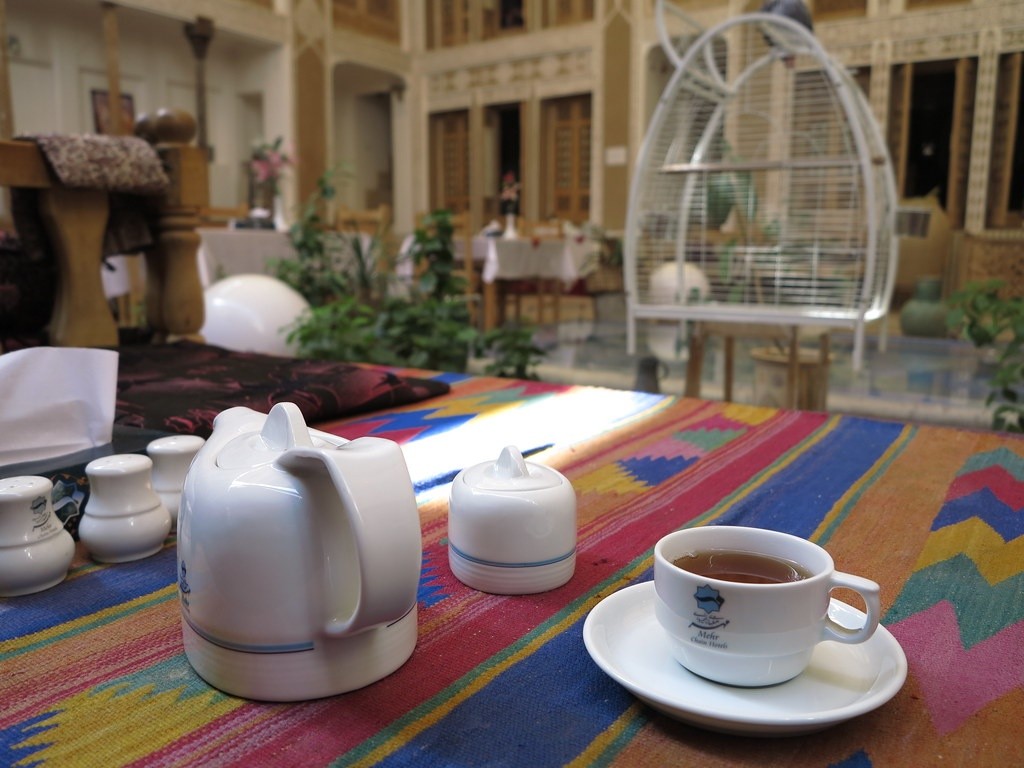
[0,336,1024,768]
[401,232,606,329]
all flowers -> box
[248,139,296,188]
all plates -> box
[582,579,907,738]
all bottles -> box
[79,453,174,561]
[0,476,76,597]
[449,446,577,594]
[147,435,205,530]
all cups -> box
[652,525,879,687]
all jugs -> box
[178,402,422,702]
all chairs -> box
[413,214,601,335]
